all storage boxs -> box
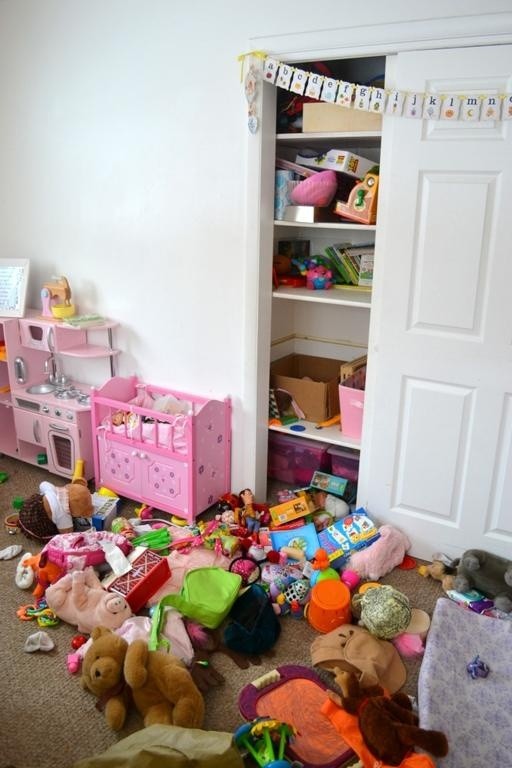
[271,351,349,423]
[265,435,360,527]
[338,366,364,436]
[302,102,383,132]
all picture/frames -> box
[0,255,29,318]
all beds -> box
[91,374,232,525]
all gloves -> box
[212,626,275,670]
[191,653,226,691]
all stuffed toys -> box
[23,551,134,636]
[79,624,206,729]
[0,55,512,768]
[334,669,448,766]
[218,488,412,655]
[426,548,512,614]
[17,477,95,545]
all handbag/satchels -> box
[153,566,243,630]
[227,584,282,652]
[311,624,406,695]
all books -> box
[328,243,375,286]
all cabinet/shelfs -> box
[245,13,512,573]
[13,407,45,447]
[56,318,122,357]
[0,307,54,472]
